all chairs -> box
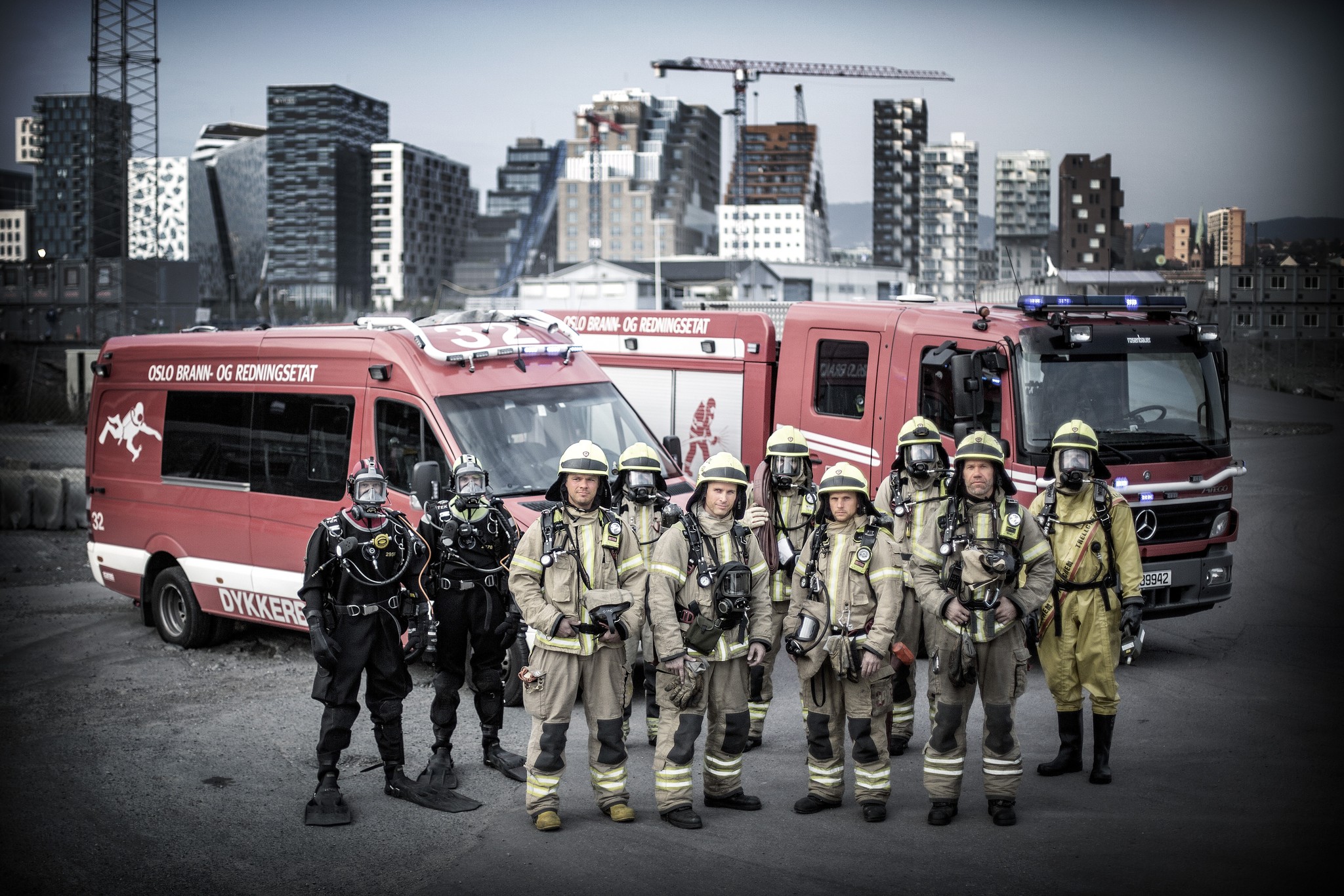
[497,404,547,475]
[1076,360,1125,428]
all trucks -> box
[474,290,1249,646]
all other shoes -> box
[648,736,657,746]
[742,739,762,752]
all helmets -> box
[449,455,486,496]
[546,440,613,510]
[1044,419,1112,482]
[611,442,668,495]
[348,458,388,510]
[814,463,881,525]
[946,431,1018,496]
[765,426,814,492]
[891,415,950,472]
[685,452,747,520]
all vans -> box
[84,309,701,651]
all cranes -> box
[651,57,955,207]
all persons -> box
[654,447,771,829]
[906,429,1056,825]
[862,409,955,756]
[776,460,904,823]
[506,439,646,832]
[288,455,483,826]
[732,426,826,751]
[602,440,684,748]
[409,457,532,785]
[1024,419,1146,785]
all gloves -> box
[665,659,705,710]
[947,634,977,687]
[1023,613,1042,643]
[1118,603,1143,638]
[302,604,344,670]
[493,603,519,651]
[404,612,430,664]
[824,634,860,685]
[735,502,769,528]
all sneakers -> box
[657,792,1018,830]
[604,805,635,821]
[532,812,561,831]
[887,737,908,756]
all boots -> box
[1037,708,1116,785]
